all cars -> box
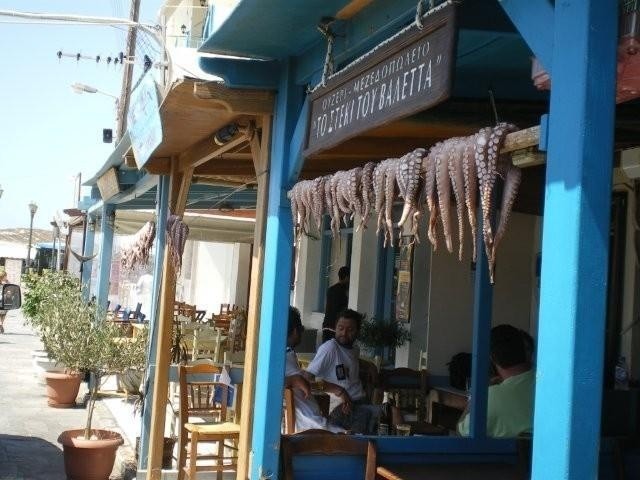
[32,245,65,270]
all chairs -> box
[280,427,402,479]
[178,362,243,479]
[280,350,448,436]
[173,299,249,365]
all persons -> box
[284,304,345,436]
[4,290,16,309]
[305,307,404,435]
[465,329,534,401]
[321,265,350,344]
[0,271,10,334]
[455,324,534,440]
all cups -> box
[396,423,412,436]
[315,376,326,393]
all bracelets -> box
[334,388,344,397]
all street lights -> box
[50,221,61,274]
[68,80,121,146]
[25,204,38,274]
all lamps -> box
[213,121,255,147]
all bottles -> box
[614,356,630,391]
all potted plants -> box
[20,268,139,479]
